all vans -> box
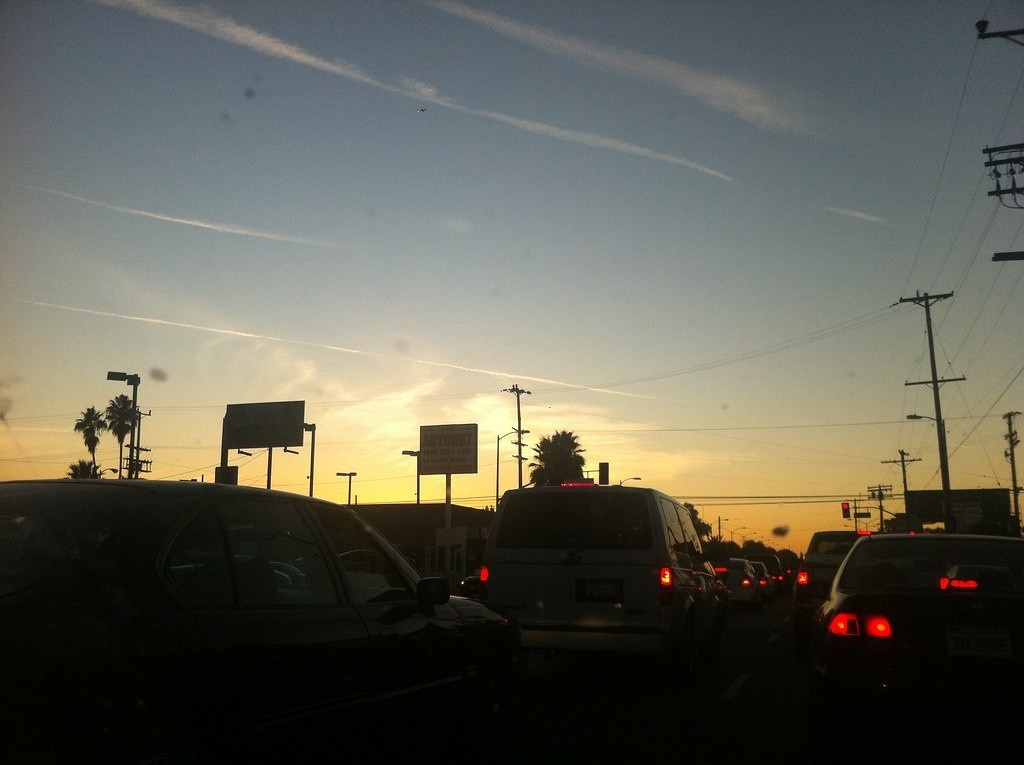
[478,485,721,654]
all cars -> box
[0,477,521,765]
[808,532,1024,765]
[714,550,798,604]
[791,532,879,620]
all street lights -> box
[618,476,640,486]
[906,414,953,536]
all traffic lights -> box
[842,502,850,518]
